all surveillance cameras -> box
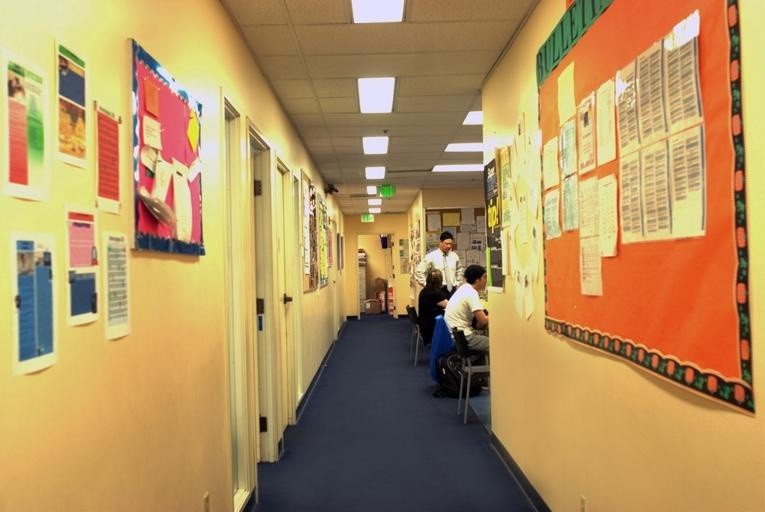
[325,184,339,195]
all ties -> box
[443,254,452,293]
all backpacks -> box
[431,351,482,399]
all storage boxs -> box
[364,299,381,314]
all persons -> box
[444,265,489,352]
[418,268,448,345]
[416,231,462,299]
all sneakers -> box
[480,378,488,391]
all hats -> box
[464,264,486,282]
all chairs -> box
[452,327,491,424]
[406,305,423,366]
[430,314,491,394]
